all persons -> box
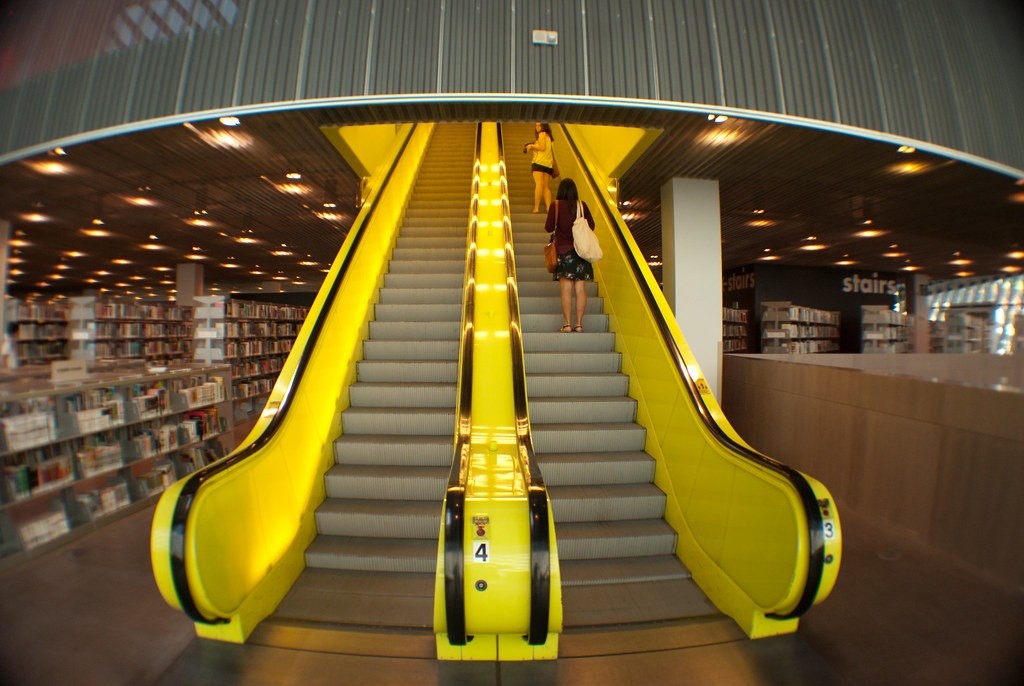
[525,121,555,212]
[545,177,595,333]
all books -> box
[723,301,993,355]
[0,294,309,553]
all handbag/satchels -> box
[572,200,603,263]
[552,160,560,178]
[544,243,557,273]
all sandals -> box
[571,326,583,332]
[560,325,572,333]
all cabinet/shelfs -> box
[760,301,840,354]
[68,296,191,370]
[0,362,234,574]
[3,298,68,369]
[0,359,148,383]
[931,307,1023,353]
[192,296,310,427]
[723,306,749,353]
[861,304,914,352]
